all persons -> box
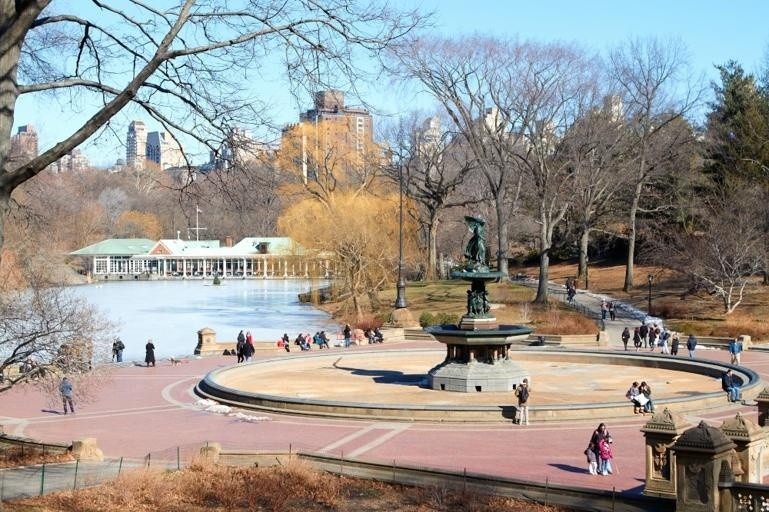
[584,423,614,475]
[18,337,94,375]
[565,277,578,304]
[729,338,741,366]
[111,338,124,362]
[600,299,608,319]
[145,338,157,368]
[466,289,491,315]
[622,320,679,355]
[280,324,384,352]
[59,376,75,417]
[629,382,643,414]
[236,330,255,363]
[514,379,532,425]
[638,381,656,413]
[686,334,697,358]
[722,370,741,402]
[609,301,617,321]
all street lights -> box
[396,138,412,309]
[647,274,653,316]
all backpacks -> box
[520,385,529,403]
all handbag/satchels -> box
[626,391,633,400]
